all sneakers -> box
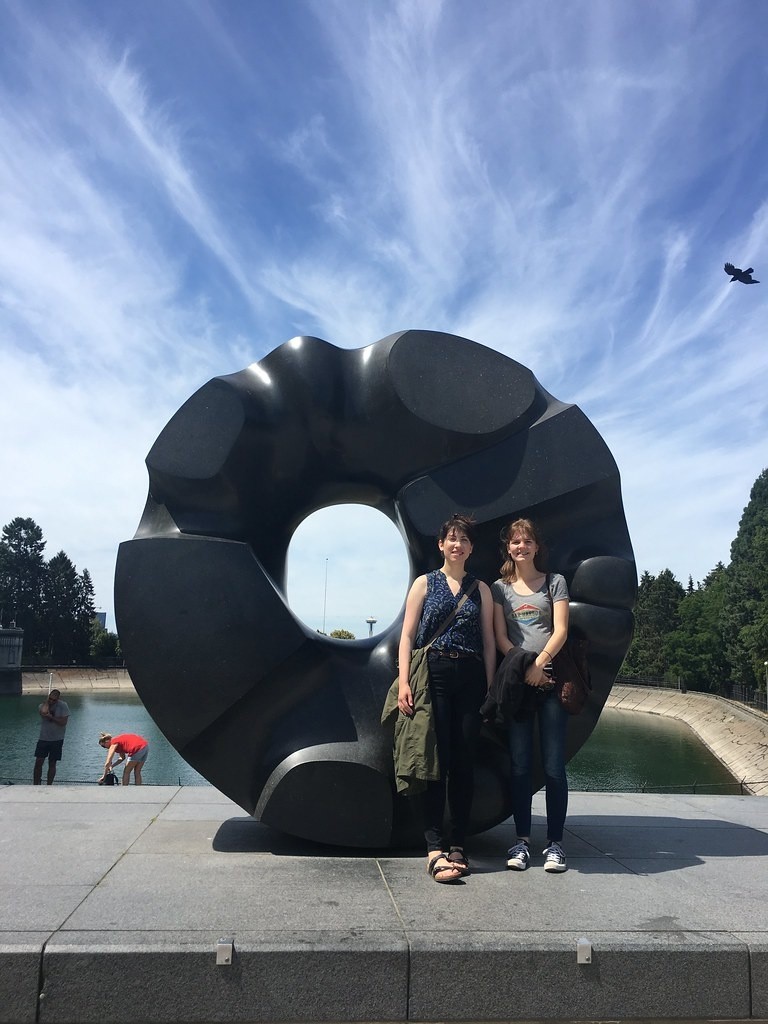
[543,841,568,872]
[505,838,532,870]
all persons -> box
[33,689,72,786]
[98,732,149,786]
[491,519,572,872]
[395,515,497,883]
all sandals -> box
[447,849,470,876]
[424,854,462,883]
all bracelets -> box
[543,650,553,659]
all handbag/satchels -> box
[100,770,119,786]
[554,623,592,715]
